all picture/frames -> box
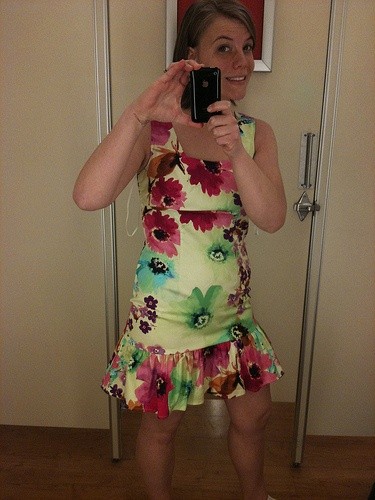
[165,1,277,73]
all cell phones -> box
[189,66,221,124]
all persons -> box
[73,1,290,500]
